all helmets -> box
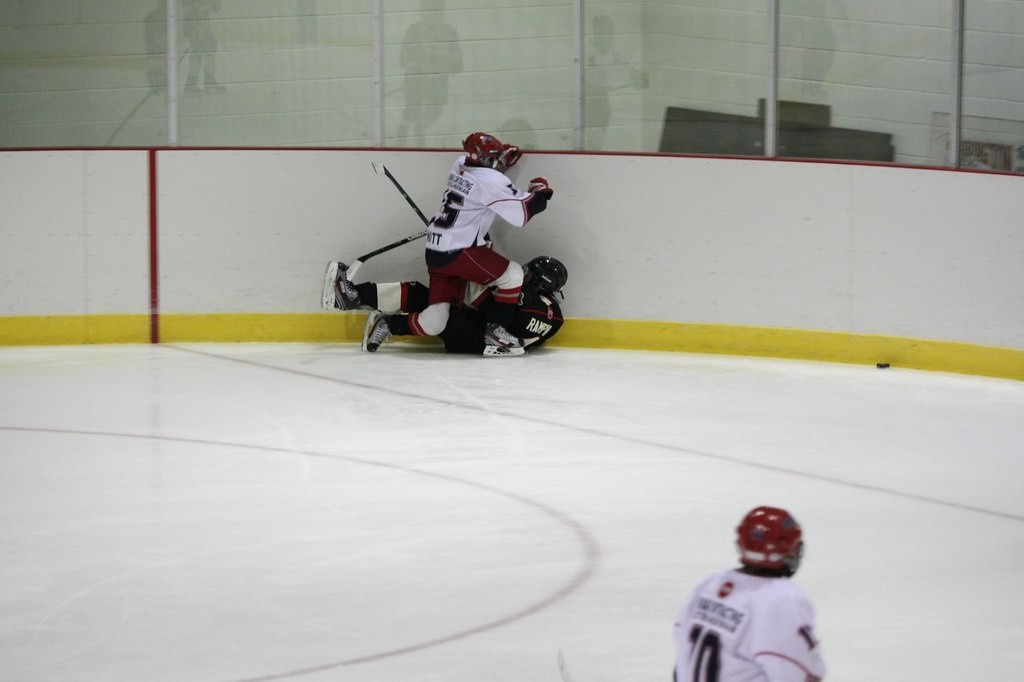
[522,256,568,294]
[462,131,502,168]
[736,506,802,569]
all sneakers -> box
[361,309,392,352]
[483,322,526,357]
[321,260,361,310]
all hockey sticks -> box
[346,161,428,282]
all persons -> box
[362,132,554,358]
[320,255,568,357]
[668,504,828,681]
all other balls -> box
[877,363,889,369]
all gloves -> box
[528,177,549,192]
[499,146,524,171]
